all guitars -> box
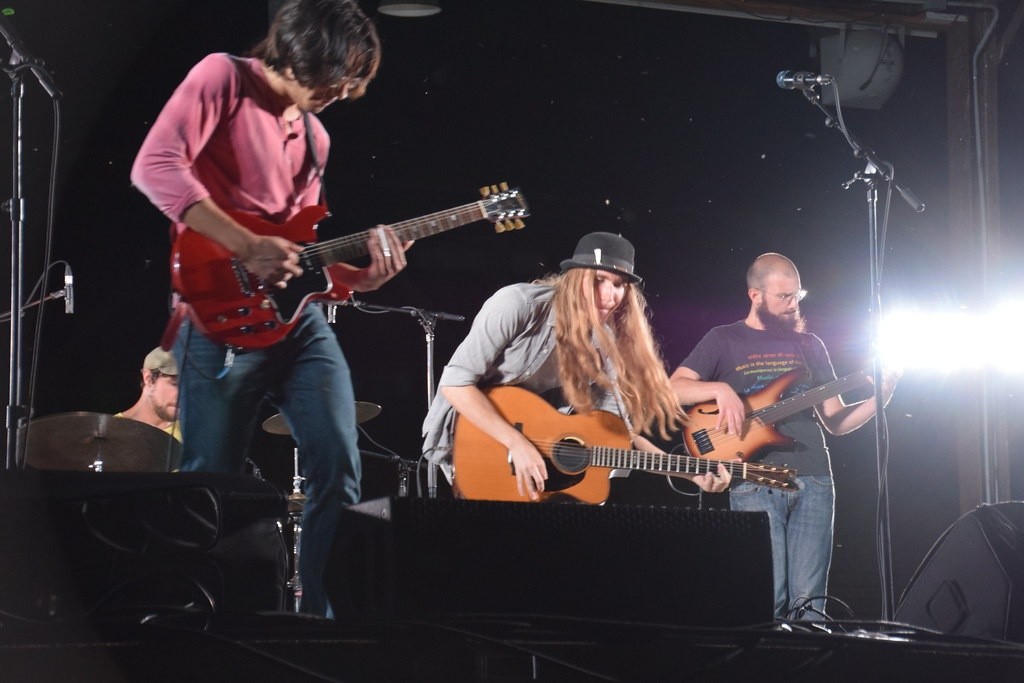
[451,384,801,506]
[680,360,896,467]
[170,181,533,355]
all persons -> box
[420,230,734,506]
[668,251,903,622]
[131,0,414,617]
[113,342,182,443]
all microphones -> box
[65,265,74,313]
[328,304,336,324]
[776,71,834,88]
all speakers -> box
[323,496,775,633]
[0,467,289,639]
[886,502,1024,643]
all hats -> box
[144,346,177,374]
[560,231,642,282]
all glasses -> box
[755,288,807,302]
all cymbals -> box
[285,493,309,511]
[261,401,384,435]
[17,410,183,474]
